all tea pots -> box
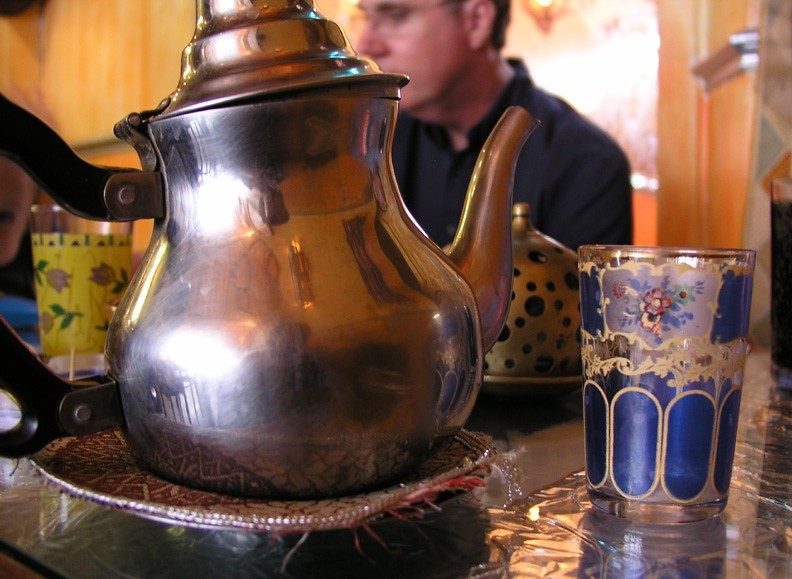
[441,204,581,380]
[0,0,542,503]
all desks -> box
[0,343,792,579]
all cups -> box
[577,244,757,528]
[30,200,136,381]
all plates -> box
[478,374,583,407]
[27,422,496,533]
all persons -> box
[239,323,329,422]
[352,0,634,259]
